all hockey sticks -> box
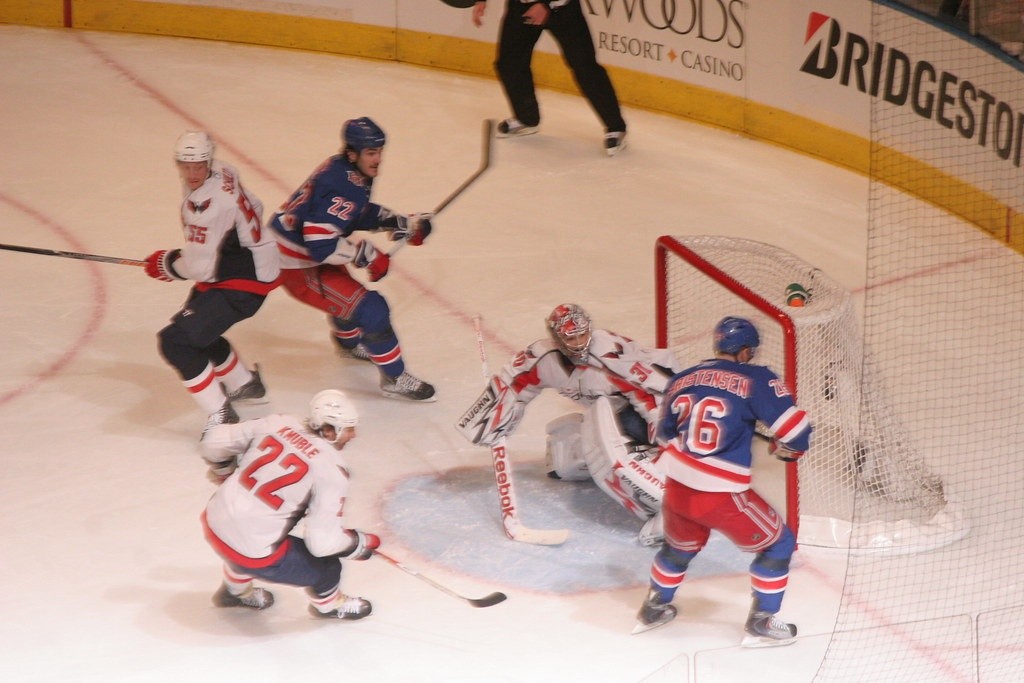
[0,245,150,267]
[471,314,570,546]
[543,317,771,442]
[372,551,507,609]
[366,118,495,274]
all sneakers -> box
[305,592,374,622]
[628,588,679,634]
[343,339,373,362]
[212,370,271,407]
[493,117,539,139]
[379,370,438,403]
[195,398,244,441]
[603,127,628,156]
[209,580,276,616]
[740,608,799,648]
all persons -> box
[145,130,287,478]
[473,0,628,156]
[630,316,812,648]
[267,117,435,403]
[198,389,380,620]
[454,302,677,547]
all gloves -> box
[206,455,239,487]
[140,249,176,283]
[339,527,380,562]
[350,237,390,282]
[766,435,805,463]
[387,210,433,247]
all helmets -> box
[711,315,760,358]
[171,130,214,178]
[306,390,360,445]
[339,117,386,154]
[544,302,593,366]
